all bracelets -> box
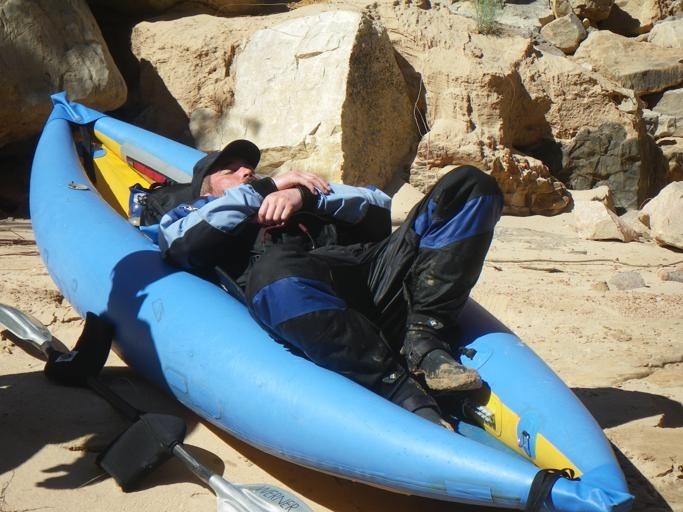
[250,175,279,198]
[295,183,315,212]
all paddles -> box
[0,304,313,512]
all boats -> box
[29,88,638,512]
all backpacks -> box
[140,181,191,226]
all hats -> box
[190,139,261,199]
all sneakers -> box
[398,327,484,394]
[388,376,456,433]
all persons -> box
[156,137,507,432]
